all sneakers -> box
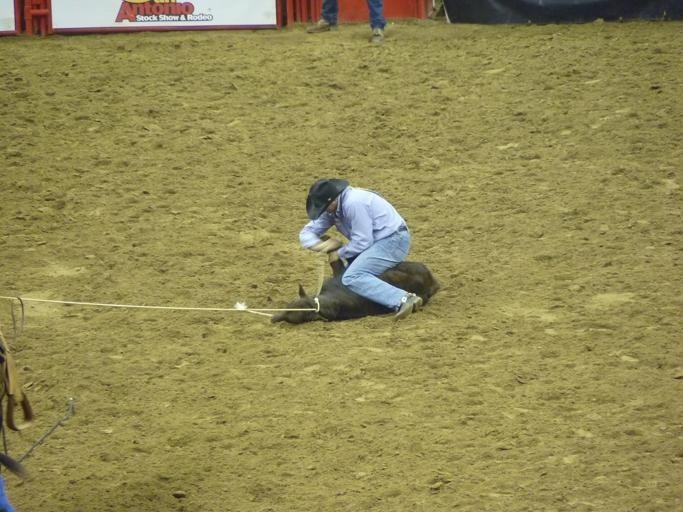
[305,20,385,42]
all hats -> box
[305,178,349,221]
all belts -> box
[388,226,408,236]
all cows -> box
[268,257,439,327]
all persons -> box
[299,179,423,321]
[305,1,386,43]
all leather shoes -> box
[392,294,424,322]
